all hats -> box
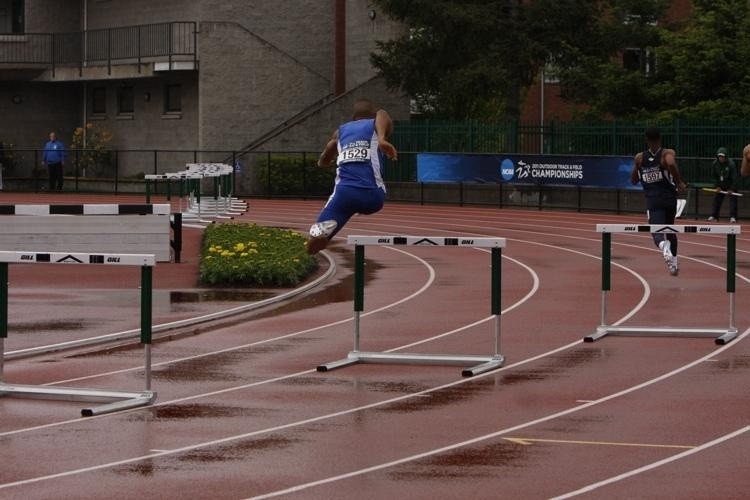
[717,153,725,156]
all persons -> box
[706,147,739,223]
[304,98,401,255]
[739,141,750,177]
[41,131,66,192]
[631,125,687,276]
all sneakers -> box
[310,219,338,238]
[663,240,680,276]
[708,216,720,221]
[730,217,736,223]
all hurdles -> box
[0,251,156,415]
[318,234,507,379]
[140,161,248,228]
[583,224,743,344]
